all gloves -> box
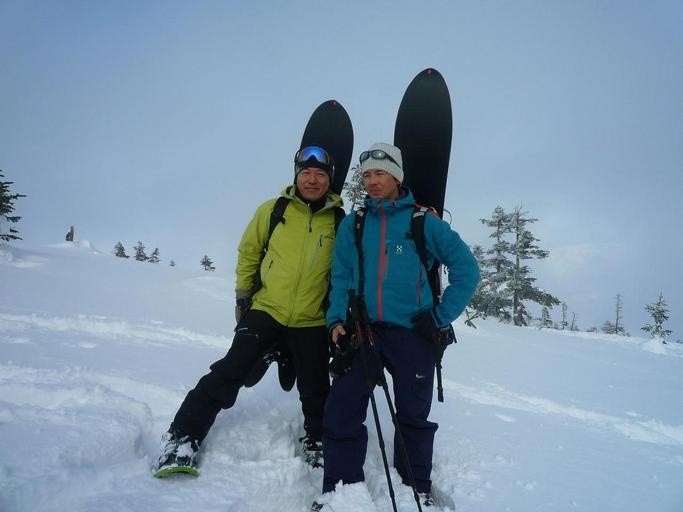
[410,309,438,338]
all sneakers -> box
[161,431,201,479]
[418,492,434,507]
[302,439,324,470]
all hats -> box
[293,142,335,184]
[360,143,405,185]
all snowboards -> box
[394,68,452,310]
[278,99,354,392]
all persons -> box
[157,145,346,468]
[310,144,476,511]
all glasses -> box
[359,149,401,168]
[294,146,335,175]
